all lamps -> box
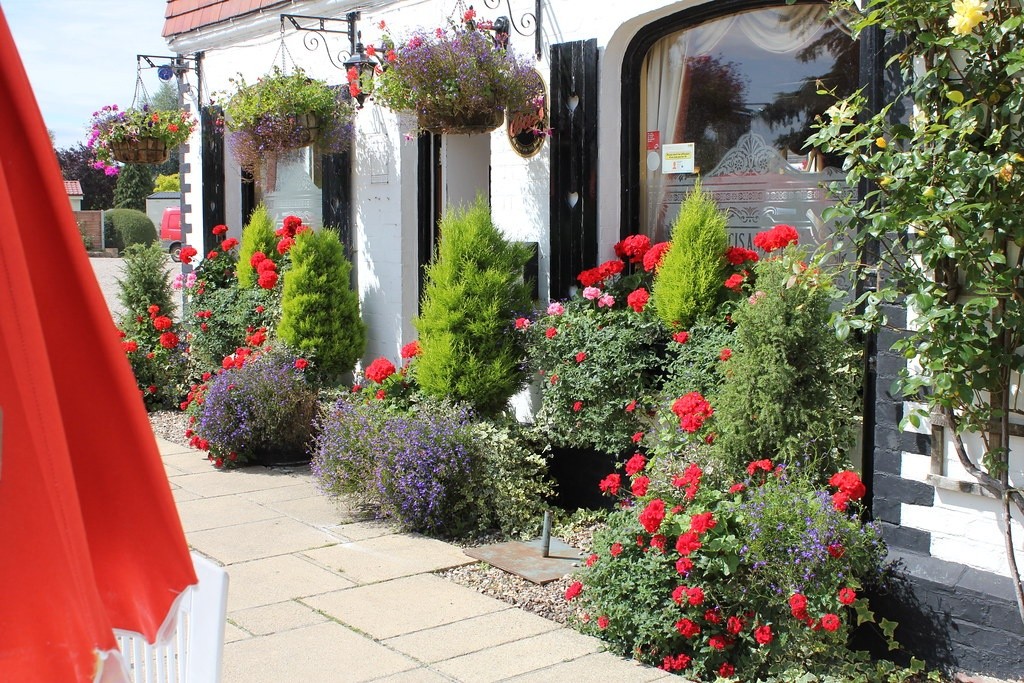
[343,31,393,107]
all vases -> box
[293,114,320,147]
[548,448,611,508]
[417,106,504,135]
[109,138,171,165]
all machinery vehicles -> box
[160,205,183,262]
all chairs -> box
[114,550,230,683]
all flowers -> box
[83,102,198,178]
[113,216,869,680]
[199,65,362,190]
[348,7,551,146]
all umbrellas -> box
[0,5,198,683]
